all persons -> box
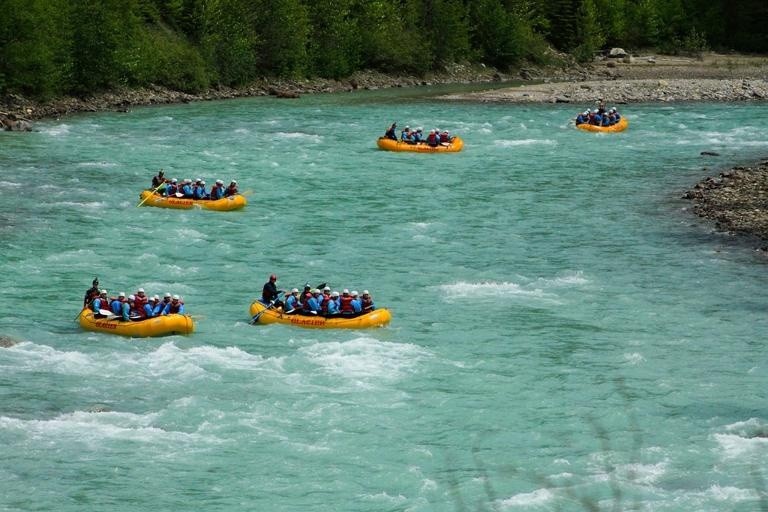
[84,278,185,322]
[575,103,620,126]
[151,169,238,201]
[262,275,378,318]
[385,123,452,147]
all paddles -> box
[249,291,284,325]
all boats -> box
[375,135,464,154]
[139,189,247,212]
[79,307,194,337]
[575,116,627,134]
[249,299,392,330]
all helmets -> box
[163,293,171,298]
[93,281,98,286]
[581,106,618,117]
[404,125,451,136]
[291,287,299,293]
[118,288,160,301]
[100,289,108,295]
[172,295,179,300]
[270,274,277,281]
[305,284,369,298]
[159,168,237,186]
